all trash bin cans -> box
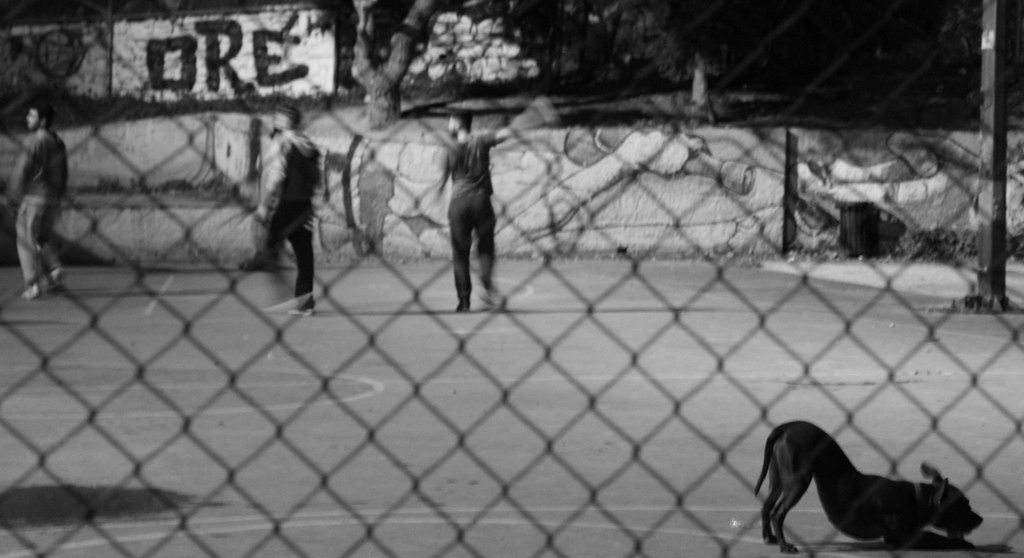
[840,201,881,256]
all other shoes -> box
[239,251,278,273]
[456,300,470,311]
[289,306,314,316]
[22,283,42,301]
[42,267,69,293]
[482,289,502,308]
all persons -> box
[239,104,319,317]
[434,110,514,311]
[9,104,68,299]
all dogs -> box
[753,420,985,554]
